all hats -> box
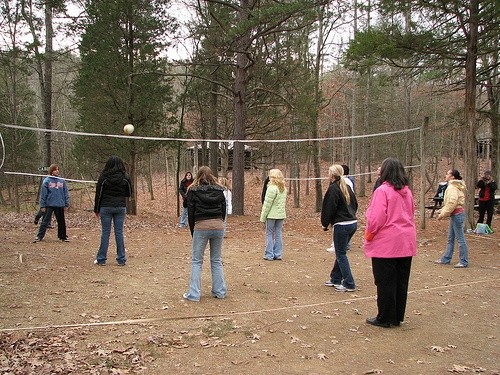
[484,170,493,175]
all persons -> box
[34,164,70,242]
[94,156,132,265]
[365,158,416,327]
[434,169,469,267]
[179,171,195,228]
[259,169,287,260]
[183,165,226,301]
[218,176,232,238]
[321,164,384,291]
[476,171,497,228]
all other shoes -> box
[116,259,126,267]
[326,243,337,253]
[93,257,107,267]
[212,292,227,300]
[61,236,70,243]
[183,292,200,302]
[33,235,41,244]
[433,258,450,265]
[454,262,467,268]
[264,254,283,261]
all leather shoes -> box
[365,316,401,328]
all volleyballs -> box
[123,124,135,134]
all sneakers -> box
[325,279,342,287]
[334,284,356,293]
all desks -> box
[429,195,500,218]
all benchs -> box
[426,205,479,209]
